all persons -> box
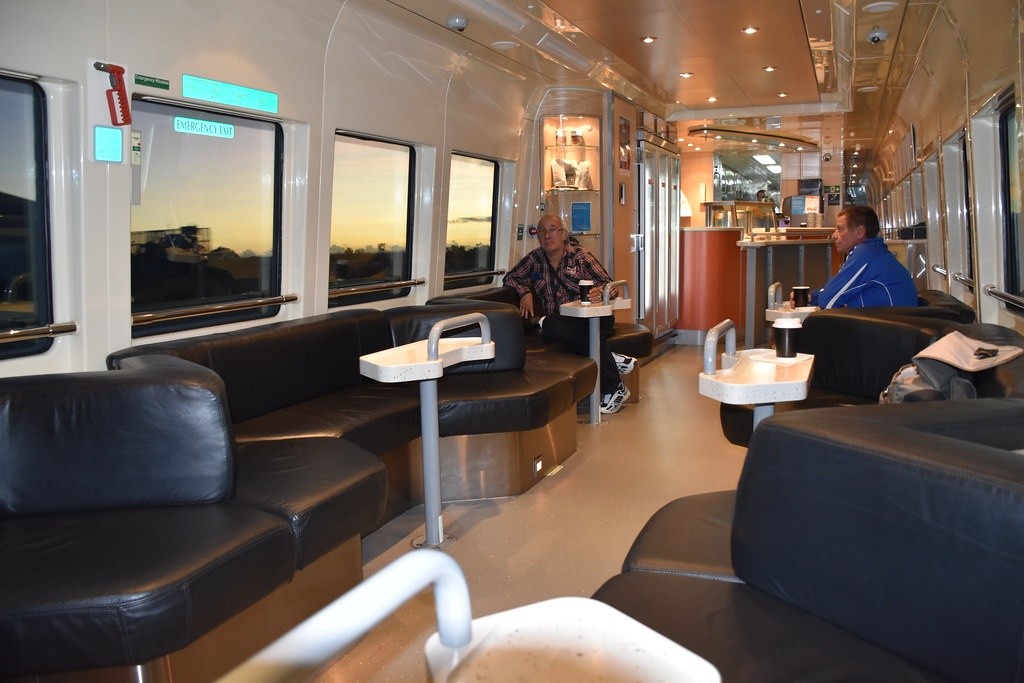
[789,205,918,309]
[757,189,777,213]
[502,214,638,414]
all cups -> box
[793,286,812,307]
[773,316,802,357]
[578,280,594,305]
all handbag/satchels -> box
[879,330,1024,405]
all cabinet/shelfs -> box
[538,112,604,269]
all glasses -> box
[536,226,563,236]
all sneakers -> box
[599,379,631,414]
[611,351,638,376]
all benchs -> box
[0,280,1024,683]
